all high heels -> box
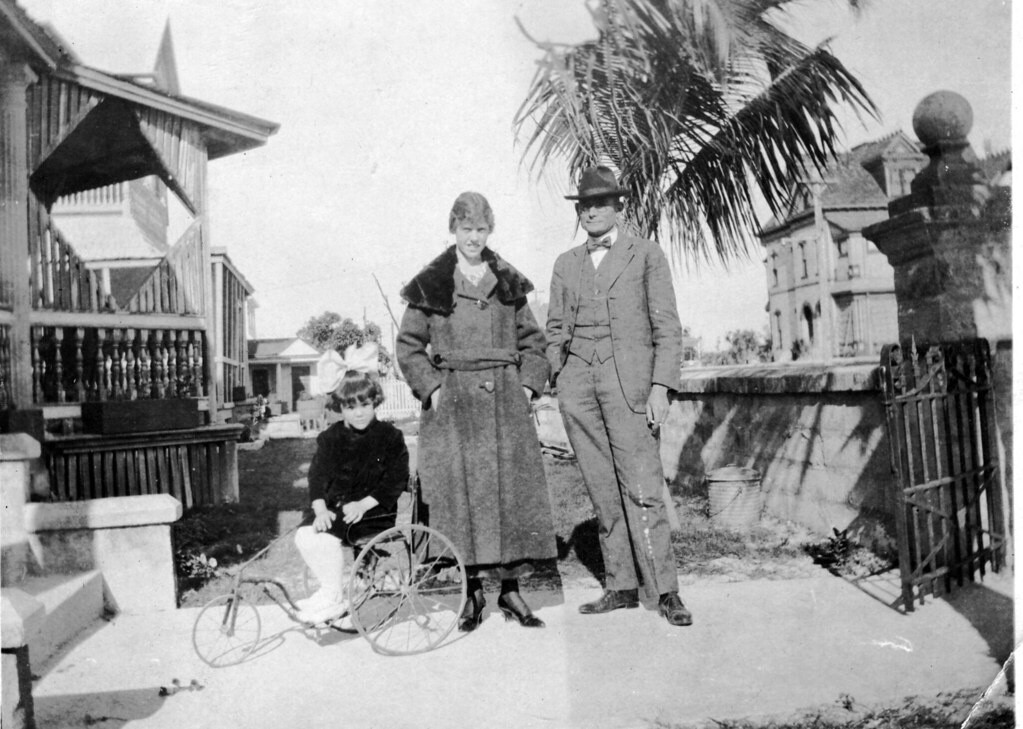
[458,596,486,631]
[497,594,544,627]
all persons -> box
[293,366,413,621]
[544,163,693,626]
[393,193,554,633]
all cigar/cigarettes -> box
[651,420,663,426]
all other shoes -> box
[328,616,364,635]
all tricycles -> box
[192,470,467,669]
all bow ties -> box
[587,236,611,250]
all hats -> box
[564,166,633,201]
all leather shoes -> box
[657,592,692,626]
[579,588,638,614]
